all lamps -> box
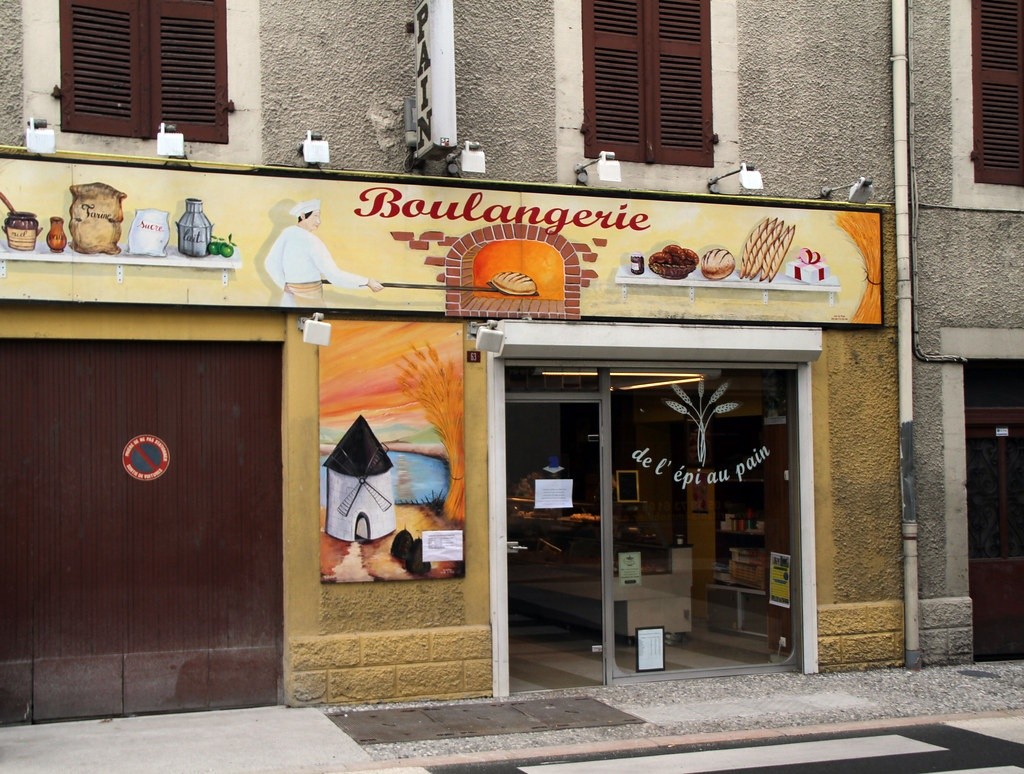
[577,150,622,185]
[298,131,330,169]
[707,163,763,195]
[156,122,184,158]
[820,176,874,206]
[25,119,58,155]
[446,141,486,176]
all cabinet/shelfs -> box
[508,521,694,645]
[705,476,771,636]
